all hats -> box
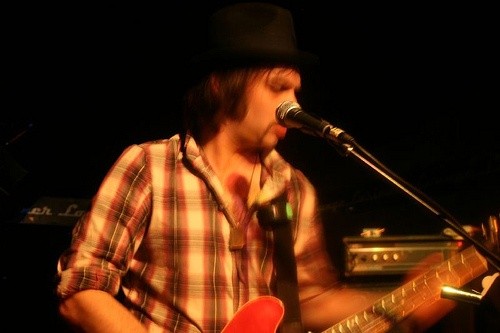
[191,3,311,78]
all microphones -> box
[275,100,355,145]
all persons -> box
[56,4,458,333]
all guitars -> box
[218,215,500,332]
